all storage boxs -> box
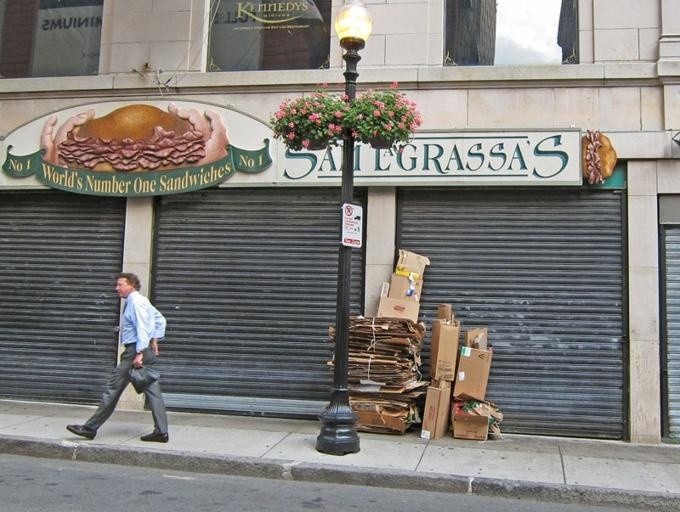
[377,248,505,441]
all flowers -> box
[269,80,349,151]
[345,80,424,140]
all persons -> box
[65,271,170,442]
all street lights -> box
[315,0,373,455]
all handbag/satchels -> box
[130,366,161,394]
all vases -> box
[370,135,393,149]
[306,139,326,150]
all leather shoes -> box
[141,433,168,442]
[67,425,96,439]
